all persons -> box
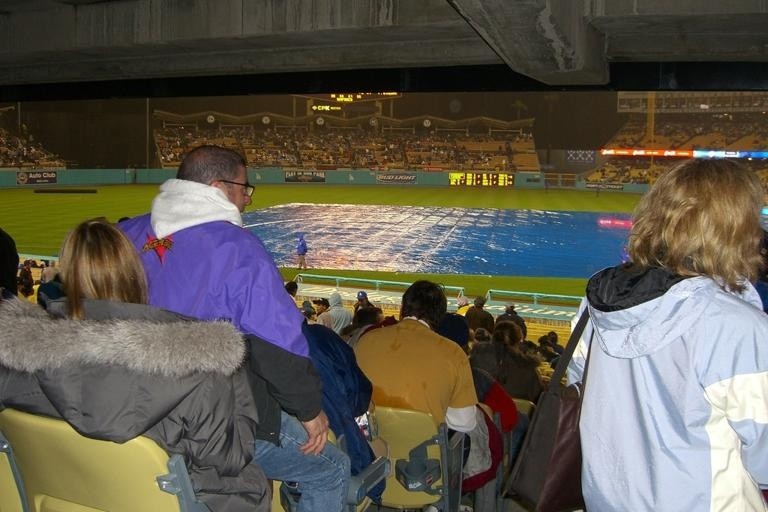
[588,94,767,204]
[564,157,766,511]
[1,215,571,511]
[102,144,352,512]
[152,113,535,176]
[1,124,62,171]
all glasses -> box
[217,180,254,196]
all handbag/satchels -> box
[501,382,585,512]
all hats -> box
[357,291,366,298]
